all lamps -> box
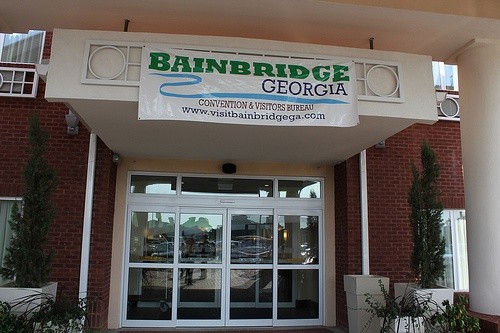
[64,107,80,137]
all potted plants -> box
[393,142,455,333]
[7,284,104,333]
[0,111,59,322]
[359,278,449,333]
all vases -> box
[343,274,390,333]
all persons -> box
[157,228,209,287]
